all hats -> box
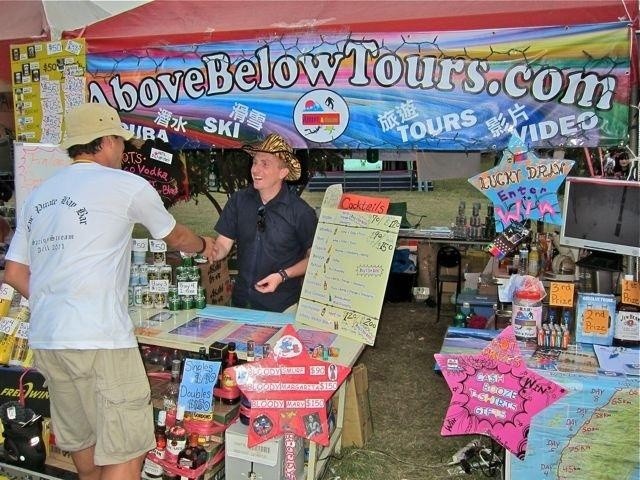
[60,103,134,149]
[243,133,302,181]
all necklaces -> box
[70,159,95,164]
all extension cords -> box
[452,438,481,464]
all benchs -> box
[309,170,434,191]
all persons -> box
[582,145,630,180]
[2,101,220,480]
[305,415,321,437]
[206,132,320,319]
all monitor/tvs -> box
[559,176,640,273]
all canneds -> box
[127,251,206,311]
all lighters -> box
[322,346,329,361]
[312,346,319,358]
[538,322,572,349]
[318,343,324,357]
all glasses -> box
[256,206,266,233]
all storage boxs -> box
[45,421,77,473]
[575,291,616,344]
[331,363,374,449]
[224,419,285,480]
[165,258,232,305]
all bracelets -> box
[196,235,206,254]
[280,269,290,284]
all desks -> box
[126,304,366,480]
[501,326,639,479]
[399,233,495,246]
[451,255,574,319]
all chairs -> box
[434,246,463,321]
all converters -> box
[466,448,475,458]
[460,459,470,472]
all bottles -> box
[513,254,519,274]
[529,247,539,277]
[143,411,168,478]
[519,243,528,276]
[163,359,181,410]
[176,432,209,480]
[221,342,241,406]
[166,405,187,456]
